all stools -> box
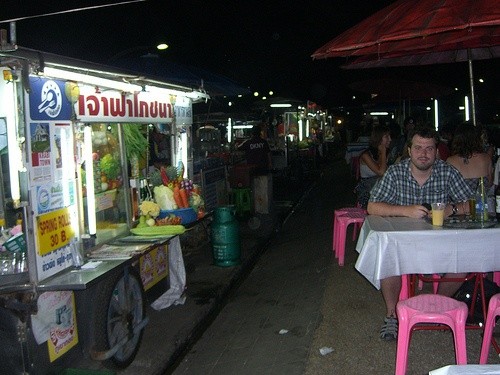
[232,188,253,218]
[479,293,500,364]
[333,208,367,266]
[395,294,468,375]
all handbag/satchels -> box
[451,276,500,326]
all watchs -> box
[449,204,458,217]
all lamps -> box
[83,122,97,239]
[36,66,199,98]
[4,80,20,207]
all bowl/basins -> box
[155,206,197,224]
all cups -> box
[430,203,445,226]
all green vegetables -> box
[109,122,151,159]
[100,154,120,178]
[189,184,205,212]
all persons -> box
[238,122,271,176]
[277,116,284,133]
[312,127,324,174]
[358,121,500,344]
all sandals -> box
[379,309,398,341]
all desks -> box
[355,215,500,358]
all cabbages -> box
[154,185,178,210]
[138,201,160,218]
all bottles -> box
[494,148,500,224]
[0,252,28,275]
[474,176,488,223]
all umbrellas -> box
[310,0,500,133]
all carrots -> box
[174,187,189,208]
